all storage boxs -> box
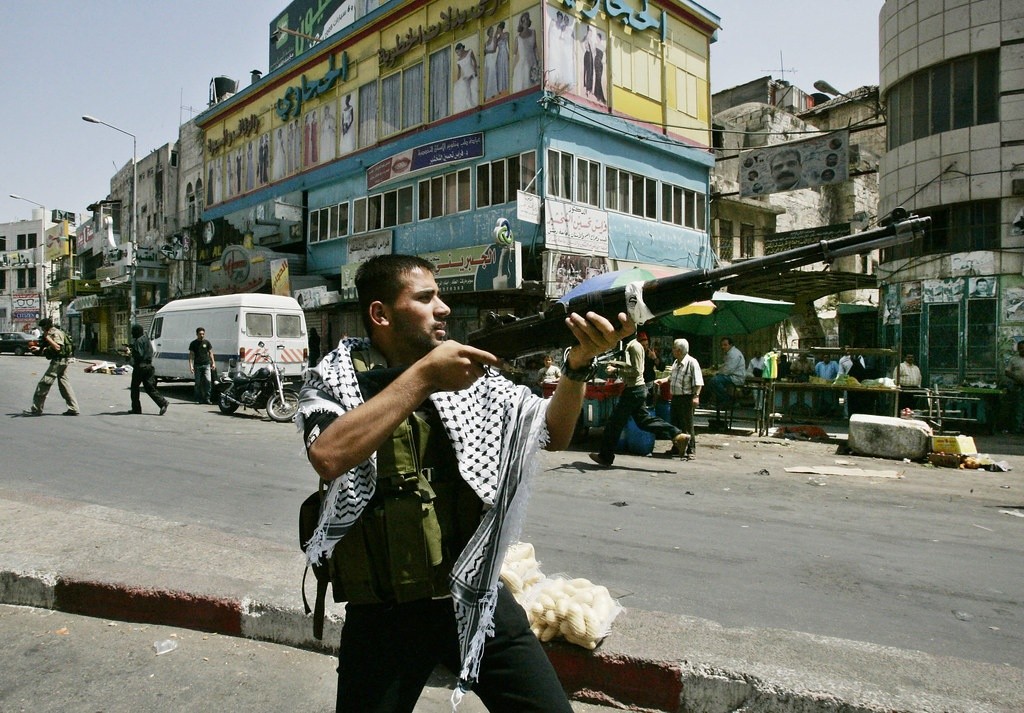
[932,434,979,457]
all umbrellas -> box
[640,291,796,372]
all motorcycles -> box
[213,340,300,423]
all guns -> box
[598,347,623,368]
[107,343,132,362]
[356,203,936,403]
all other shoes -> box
[665,446,678,457]
[686,453,695,460]
[159,401,170,415]
[128,409,142,414]
[672,432,692,458]
[589,454,611,467]
[23,409,41,417]
[201,398,213,404]
[63,410,80,416]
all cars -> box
[0,331,42,356]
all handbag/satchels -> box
[298,473,329,645]
[64,335,75,355]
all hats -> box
[131,324,144,338]
[39,318,53,326]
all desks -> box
[542,380,671,429]
[950,386,1007,432]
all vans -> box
[130,293,309,402]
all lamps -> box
[157,244,208,262]
[814,80,887,117]
[272,26,322,42]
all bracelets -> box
[694,393,699,396]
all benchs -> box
[725,382,769,435]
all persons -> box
[127,325,169,416]
[25,318,80,417]
[189,326,217,405]
[652,339,705,460]
[298,254,628,713]
[451,9,605,113]
[637,334,665,382]
[537,354,563,399]
[206,91,354,205]
[308,326,320,367]
[743,138,841,192]
[893,352,922,410]
[1003,340,1024,436]
[707,337,747,404]
[748,344,867,418]
[969,278,992,298]
[590,328,691,469]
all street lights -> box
[82,115,137,327]
[10,194,46,317]
